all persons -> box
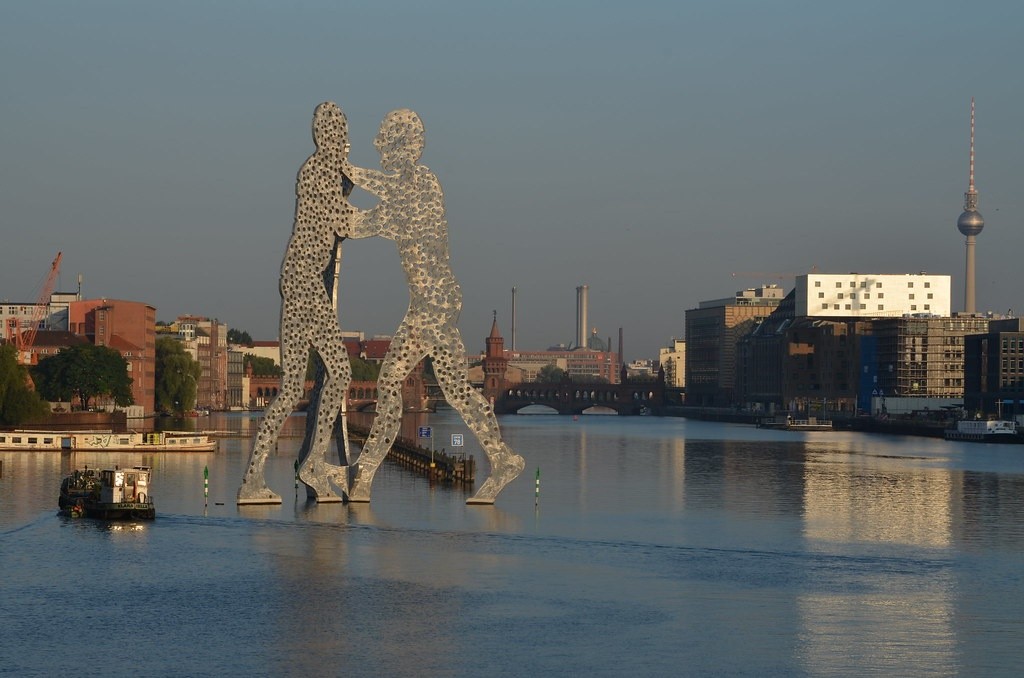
[324,109,524,505]
[238,102,379,504]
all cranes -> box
[5,252,63,394]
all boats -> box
[58,466,155,520]
[942,420,1019,443]
